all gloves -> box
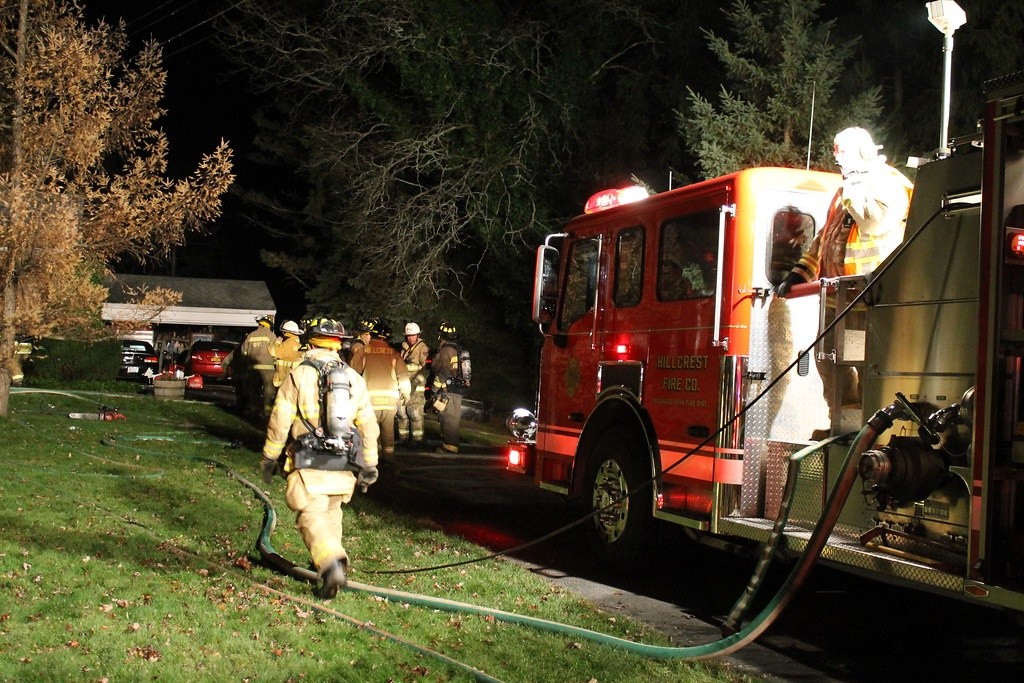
[260,457,278,484]
[356,465,380,493]
[410,378,419,395]
[400,392,410,406]
[777,271,807,298]
[840,165,859,180]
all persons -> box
[265,319,381,599]
[242,320,306,419]
[778,128,913,443]
[349,319,463,467]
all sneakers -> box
[315,559,345,598]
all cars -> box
[117,339,160,384]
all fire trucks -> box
[504,0,1024,617]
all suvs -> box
[181,340,235,382]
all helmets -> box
[279,321,305,335]
[369,323,391,342]
[359,317,376,336]
[436,320,459,339]
[833,126,875,149]
[255,314,279,328]
[402,322,423,335]
[302,316,354,341]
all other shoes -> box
[381,456,391,467]
[407,441,421,448]
[435,446,454,454]
[397,437,405,444]
